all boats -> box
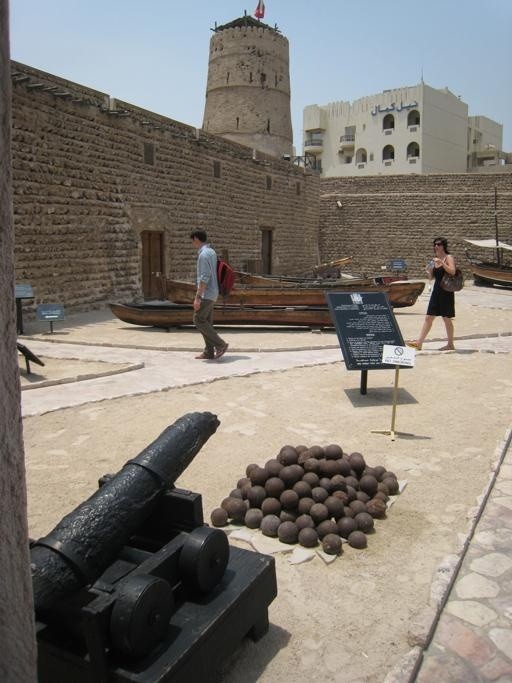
[462,235,512,288]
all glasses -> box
[434,242,442,246]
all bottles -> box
[428,259,435,268]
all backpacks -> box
[216,255,236,299]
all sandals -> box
[439,344,455,351]
[404,340,422,350]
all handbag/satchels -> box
[439,269,464,292]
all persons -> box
[190,228,229,361]
[405,236,456,351]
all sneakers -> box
[215,343,229,359]
[195,353,214,359]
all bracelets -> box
[196,294,202,299]
